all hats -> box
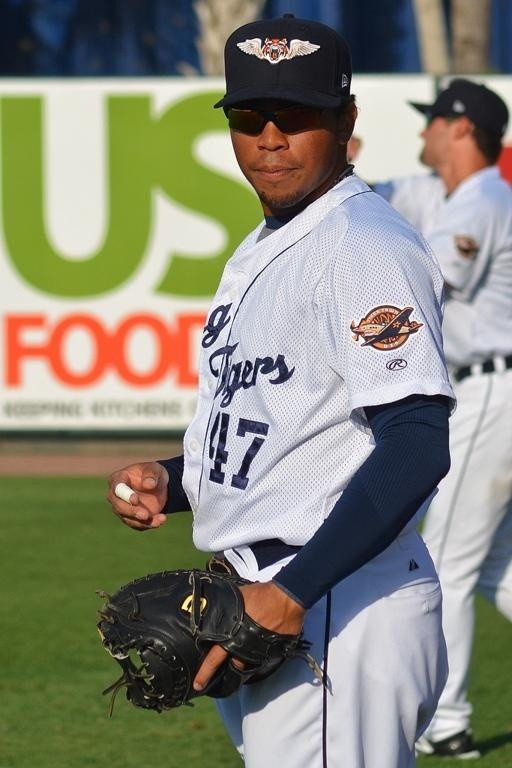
[213,11,353,112]
[406,77,508,140]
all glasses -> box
[224,105,332,136]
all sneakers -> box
[410,728,481,762]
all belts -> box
[205,538,303,580]
[454,352,512,381]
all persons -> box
[94,12,459,768]
[386,76,512,760]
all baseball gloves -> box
[95,568,327,718]
